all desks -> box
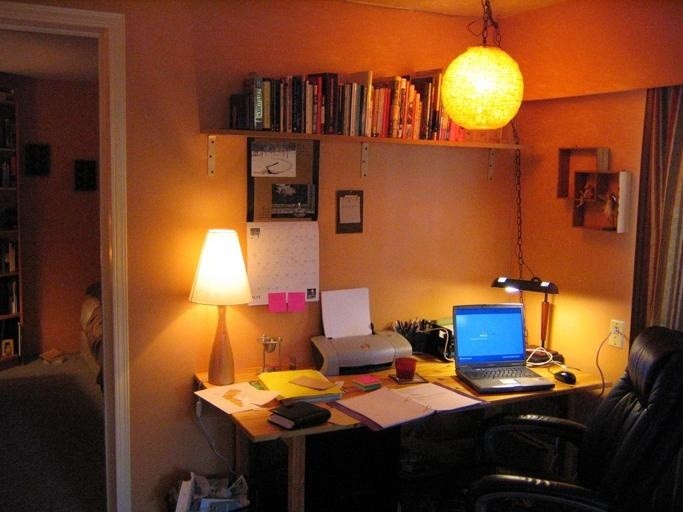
[183,348,619,511]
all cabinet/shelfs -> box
[551,143,632,235]
[0,87,30,371]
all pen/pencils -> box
[393,318,433,332]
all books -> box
[228,70,504,143]
[443,324,454,353]
[0,103,20,360]
[250,369,487,431]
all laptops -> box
[451,303,556,392]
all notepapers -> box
[352,376,381,391]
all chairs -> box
[452,321,683,511]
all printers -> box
[310,324,415,376]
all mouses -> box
[554,370,576,385]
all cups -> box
[393,357,417,379]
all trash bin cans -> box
[170,474,252,512]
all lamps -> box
[491,275,567,367]
[185,227,255,385]
[437,1,527,131]
